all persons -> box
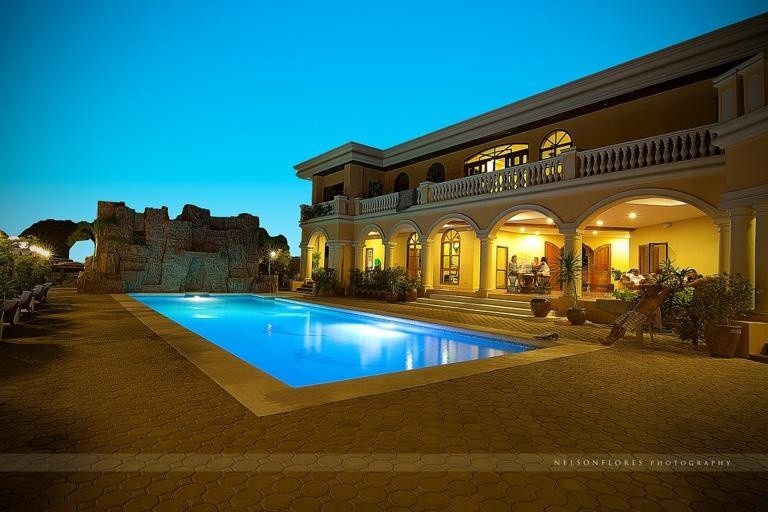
[530,256,550,286]
[509,255,521,293]
[620,269,646,286]
[598,285,668,346]
[683,269,703,283]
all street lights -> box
[269,252,276,292]
[8,236,49,295]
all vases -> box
[530,298,550,317]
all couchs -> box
[619,273,661,293]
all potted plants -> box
[699,270,754,356]
[546,249,587,324]
[402,278,421,301]
[384,279,401,303]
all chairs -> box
[515,271,551,295]
[1,281,55,338]
[621,307,659,348]
